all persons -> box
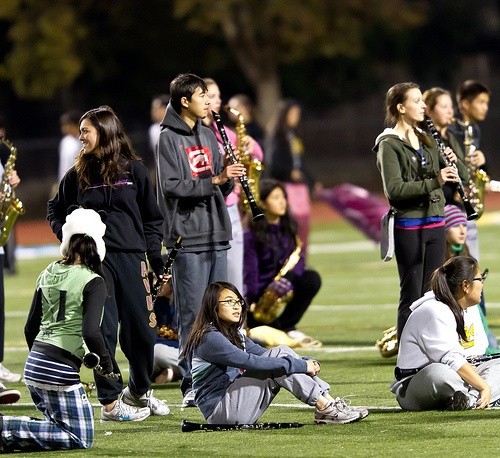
[46,104,172,422]
[183,282,370,425]
[372,80,491,351]
[390,254,500,410]
[0,204,114,454]
[0,121,23,405]
[151,73,321,385]
[59,110,83,183]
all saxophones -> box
[377,324,398,358]
[227,107,265,213]
[448,117,490,221]
[0,136,26,247]
[250,234,304,322]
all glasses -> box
[473,274,483,281]
[218,299,244,306]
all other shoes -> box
[0,362,22,382]
[0,382,20,405]
[288,330,311,343]
[155,369,178,384]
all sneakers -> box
[335,395,370,419]
[181,390,197,407]
[485,398,500,409]
[446,391,477,411]
[120,386,170,415]
[314,401,362,424]
[100,399,151,423]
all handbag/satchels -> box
[380,211,395,262]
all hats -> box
[60,206,108,262]
[443,204,468,230]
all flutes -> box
[181,418,305,432]
[394,353,500,381]
[211,109,264,220]
[82,352,120,381]
[153,233,183,299]
[423,114,479,221]
